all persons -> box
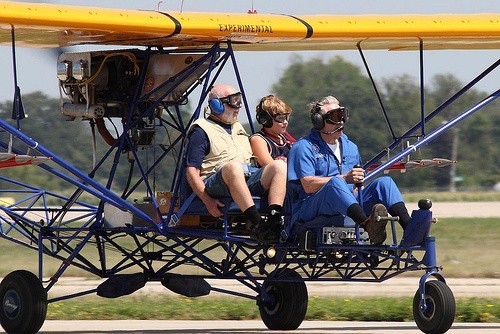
[249,95,296,166]
[288,96,411,245]
[182,84,287,243]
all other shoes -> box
[251,224,275,243]
[367,204,388,244]
[266,221,286,233]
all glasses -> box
[272,113,291,123]
[325,112,348,125]
[219,92,243,108]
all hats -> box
[313,96,345,116]
[209,85,239,100]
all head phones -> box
[256,101,273,128]
[209,98,225,114]
[311,100,325,131]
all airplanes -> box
[0,2,500,334]
